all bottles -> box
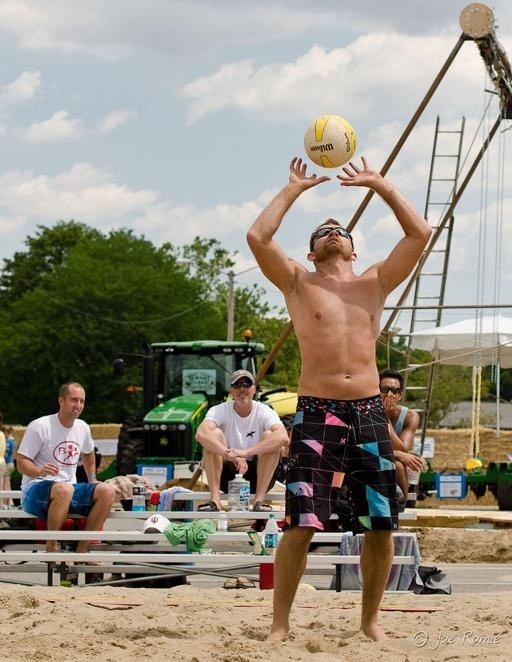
[133,480,146,511]
[218,510,227,534]
[265,513,279,555]
[227,473,252,512]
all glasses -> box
[232,380,256,389]
[310,227,354,252]
[379,387,401,393]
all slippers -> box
[254,501,272,512]
[223,576,255,588]
[198,500,219,511]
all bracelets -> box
[86,472,98,479]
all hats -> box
[142,514,170,534]
[231,369,255,386]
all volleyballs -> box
[304,115,357,168]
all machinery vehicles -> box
[108,327,299,512]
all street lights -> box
[174,257,260,390]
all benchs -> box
[0,484,434,591]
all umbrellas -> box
[409,312,512,438]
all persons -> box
[0,410,18,515]
[193,368,290,514]
[16,379,116,567]
[379,369,423,507]
[244,156,435,645]
